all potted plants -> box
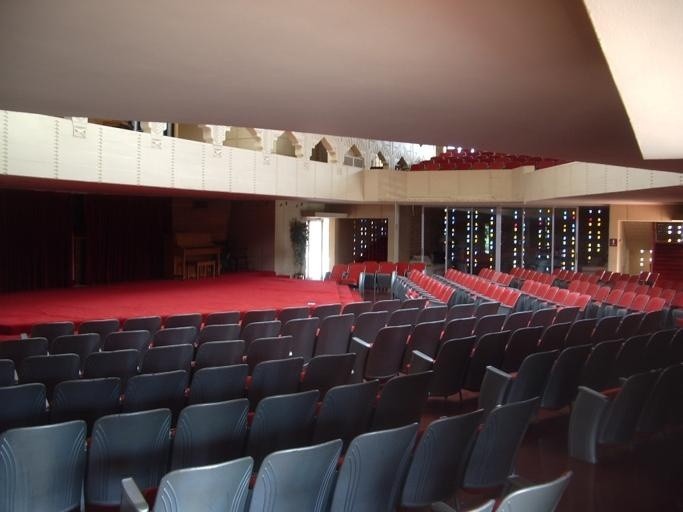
[289,217,311,280]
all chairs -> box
[408,149,560,172]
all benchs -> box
[188,260,217,280]
[226,256,250,273]
